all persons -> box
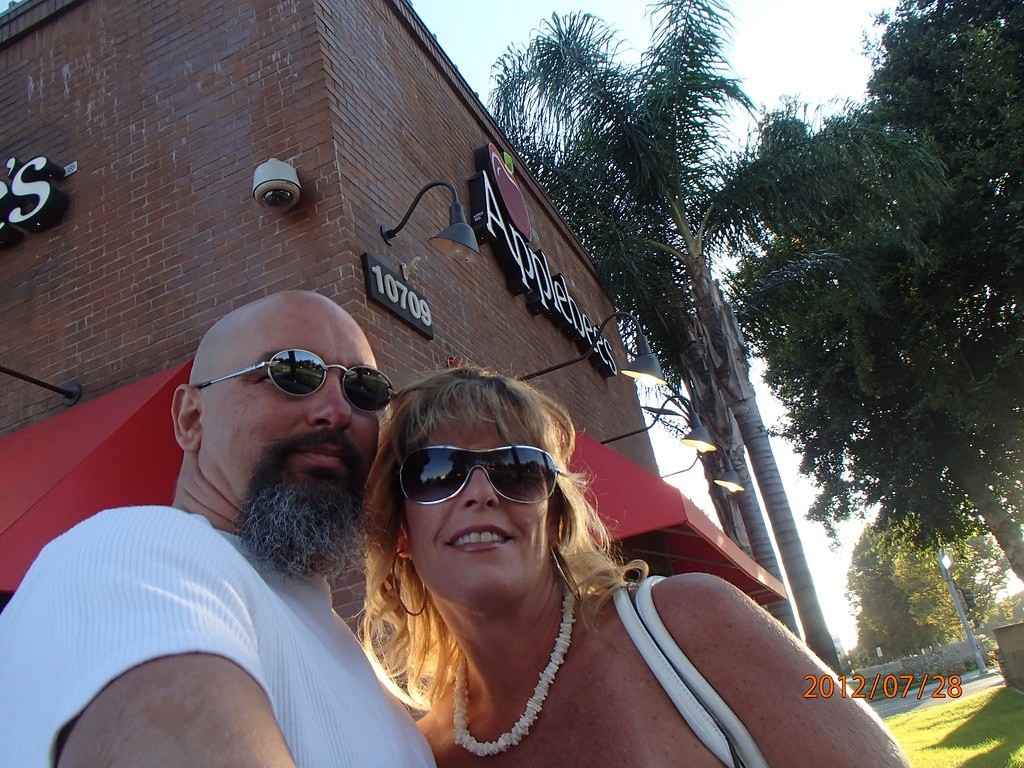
[364,359,919,767]
[1,287,451,768]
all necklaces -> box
[452,575,574,756]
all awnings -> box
[0,358,199,595]
[566,430,789,605]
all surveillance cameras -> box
[252,158,302,212]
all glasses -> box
[194,349,397,413]
[399,446,562,506]
